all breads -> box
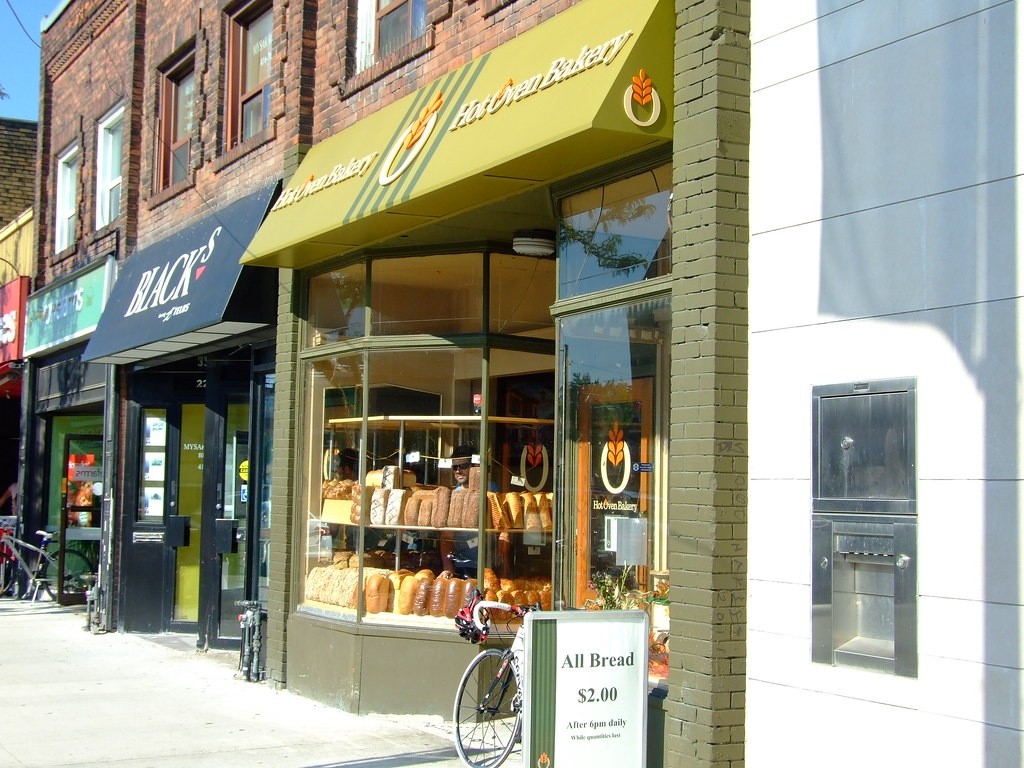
[321,468,553,530]
[306,550,551,622]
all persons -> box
[436,445,499,580]
[330,448,387,551]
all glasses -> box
[335,464,342,469]
[452,462,472,471]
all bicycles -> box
[0,519,95,601]
[452,598,542,767]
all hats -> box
[334,449,359,473]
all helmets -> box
[454,606,491,644]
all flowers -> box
[583,561,656,612]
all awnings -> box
[241,0,673,267]
[81,181,282,366]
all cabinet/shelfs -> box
[305,414,554,534]
